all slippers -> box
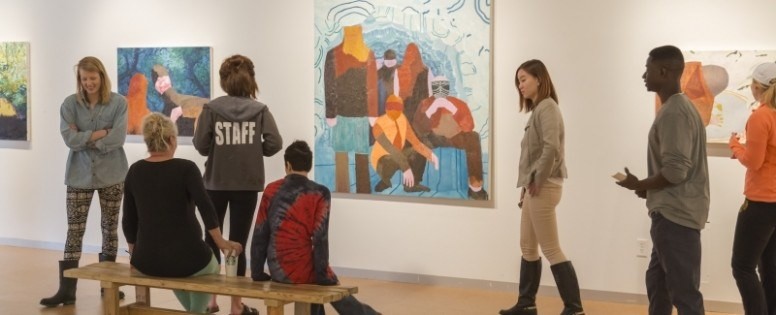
[228,302,259,315]
[206,305,219,315]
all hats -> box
[746,61,776,87]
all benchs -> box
[63,262,360,315]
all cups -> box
[224,248,239,278]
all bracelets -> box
[104,128,109,135]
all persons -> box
[729,62,776,315]
[250,140,382,315]
[499,59,585,315]
[122,113,242,315]
[39,57,129,306]
[192,55,282,315]
[616,45,711,315]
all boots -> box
[98,253,126,299]
[39,260,78,307]
[499,256,543,315]
[549,259,585,315]
